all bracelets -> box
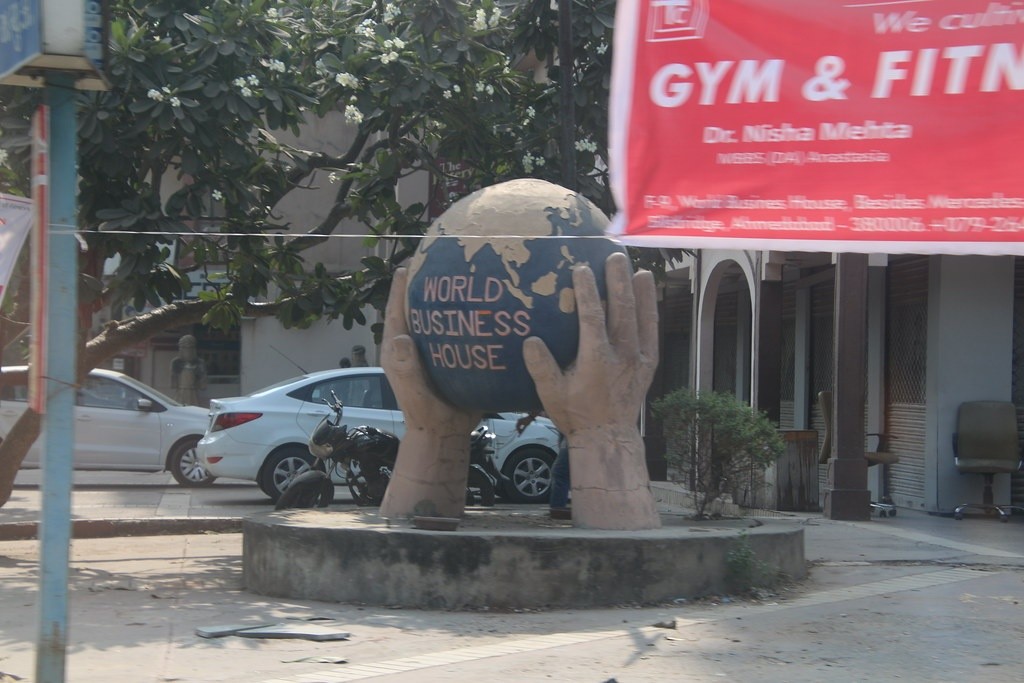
[529,414,535,420]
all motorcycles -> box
[270,388,511,511]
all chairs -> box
[951,400,1024,523]
[361,381,375,407]
[815,391,901,524]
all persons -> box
[380,252,659,531]
[516,410,570,515]
[170,334,207,407]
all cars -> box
[192,365,564,503]
[0,362,218,489]
